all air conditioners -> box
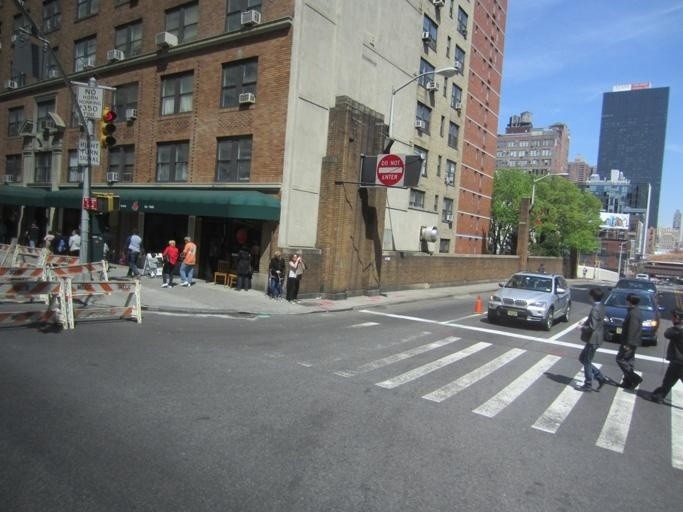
[454,61,461,69]
[241,10,261,25]
[4,80,15,88]
[415,120,425,129]
[430,81,440,90]
[432,0,445,6]
[455,103,463,110]
[106,172,119,182]
[2,175,11,181]
[238,93,255,105]
[445,215,454,221]
[422,31,432,40]
[106,49,122,61]
[126,109,137,119]
[154,31,177,45]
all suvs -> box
[487,270,571,330]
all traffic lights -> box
[101,108,115,148]
[97,196,120,212]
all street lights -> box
[615,239,637,281]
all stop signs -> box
[377,155,404,185]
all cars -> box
[588,287,665,345]
[607,274,674,299]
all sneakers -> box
[180,281,188,286]
[161,281,168,288]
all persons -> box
[574,288,609,392]
[582,267,587,278]
[161,239,179,288]
[615,293,642,390]
[537,263,545,273]
[286,254,305,304]
[26,220,81,256]
[123,229,143,279]
[650,308,683,404]
[100,225,113,261]
[178,235,198,287]
[234,240,256,290]
[267,250,285,301]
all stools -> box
[226,274,238,287]
[214,272,227,286]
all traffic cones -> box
[473,295,484,315]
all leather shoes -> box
[598,377,609,390]
[577,384,593,392]
[620,373,643,391]
